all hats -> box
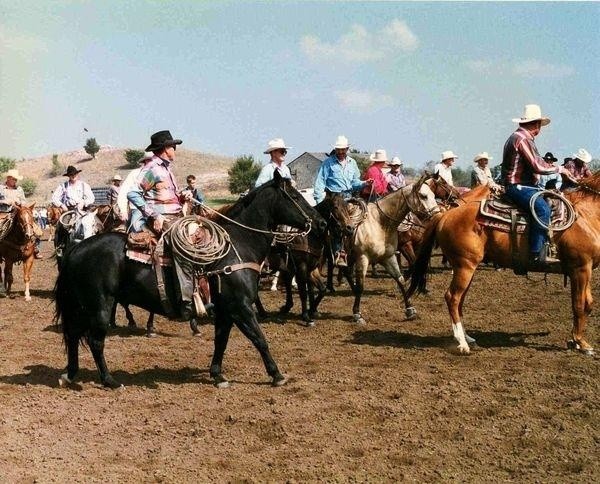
[111,174,123,181]
[387,157,402,165]
[542,152,557,161]
[5,169,23,180]
[473,152,493,162]
[139,150,154,162]
[370,150,388,162]
[329,136,354,148]
[145,130,182,152]
[440,151,458,161]
[62,166,82,176]
[573,149,591,162]
[263,139,291,154]
[561,157,574,166]
[511,104,551,126]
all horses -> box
[109,205,236,340]
[439,181,495,213]
[399,170,599,359]
[369,172,461,279]
[307,173,442,327]
[46,205,65,239]
[0,201,39,302]
[52,209,103,270]
[48,167,329,390]
[251,187,353,327]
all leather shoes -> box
[34,251,42,258]
[182,302,214,320]
[530,253,560,268]
[56,245,65,256]
[334,254,347,266]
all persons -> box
[254,139,296,191]
[434,151,458,189]
[537,153,563,191]
[178,176,203,206]
[127,130,214,322]
[53,166,95,257]
[33,209,40,224]
[313,136,374,267]
[108,175,122,205]
[502,103,566,264]
[385,158,406,193]
[471,151,502,192]
[0,169,42,260]
[361,150,388,202]
[564,150,593,187]
[119,152,156,222]
[40,207,48,230]
